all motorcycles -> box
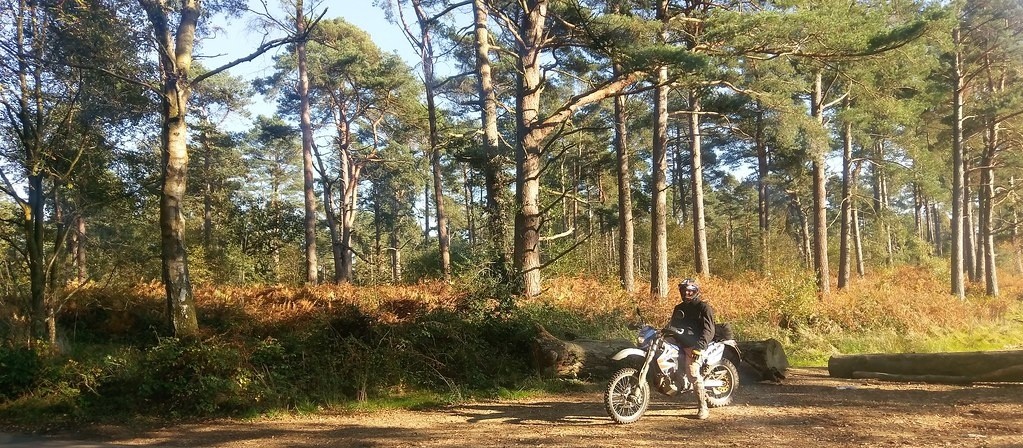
[603,308,742,425]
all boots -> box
[695,389,709,420]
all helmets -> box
[678,279,700,303]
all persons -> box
[660,279,715,420]
